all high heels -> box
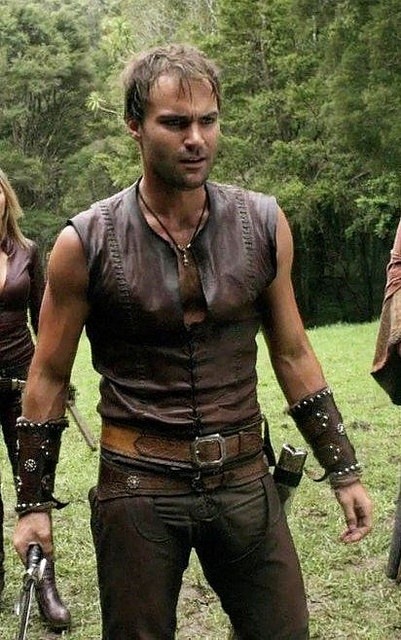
[34,562,72,628]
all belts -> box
[99,422,264,468]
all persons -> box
[0,168,76,632]
[12,43,374,640]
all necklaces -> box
[137,183,208,267]
